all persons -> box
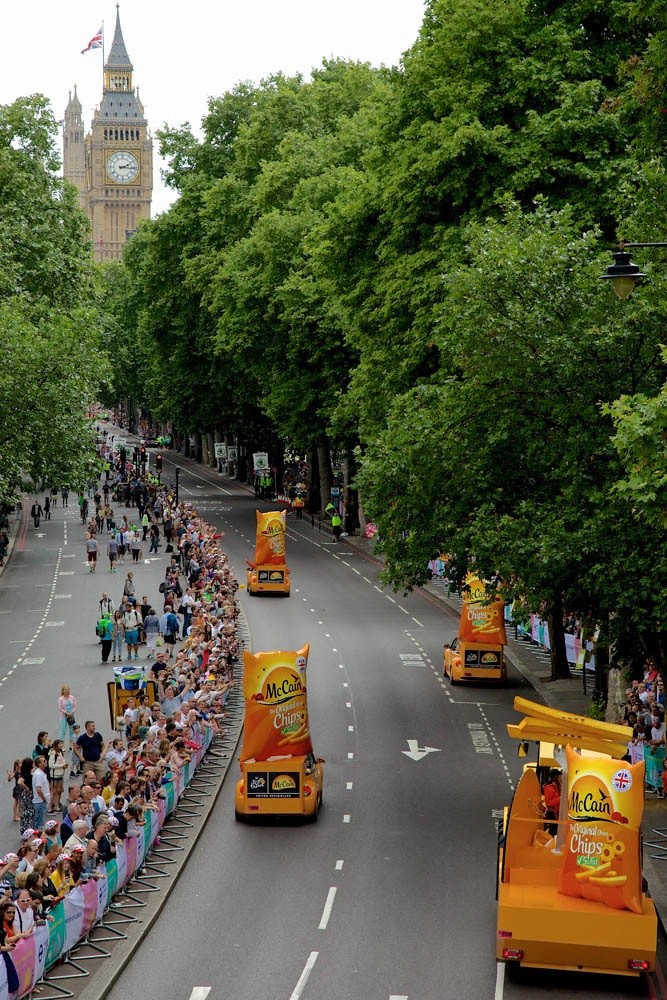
[620,658,667,799]
[1,426,240,994]
[254,471,273,500]
[292,493,305,521]
[324,506,342,543]
[544,769,563,838]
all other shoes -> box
[70,769,82,778]
[46,807,63,815]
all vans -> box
[246,566,293,597]
[444,637,508,684]
[234,753,327,820]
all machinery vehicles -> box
[493,696,657,982]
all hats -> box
[75,798,87,803]
[652,716,660,723]
[4,853,21,861]
[212,647,221,652]
[72,725,81,729]
[56,854,73,863]
[549,769,563,777]
[0,859,7,865]
[71,844,85,852]
[31,838,47,847]
[45,820,61,830]
[108,817,119,826]
[104,612,112,616]
[23,828,39,840]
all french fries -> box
[576,861,627,886]
[272,549,285,558]
[278,723,310,747]
[506,696,633,759]
[472,622,499,633]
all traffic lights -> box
[132,452,138,466]
[156,455,163,473]
[140,447,148,462]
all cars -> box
[140,435,161,447]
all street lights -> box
[175,465,181,507]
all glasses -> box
[5,911,16,914]
[19,898,32,903]
[45,866,50,869]
[657,684,664,686]
[154,709,160,712]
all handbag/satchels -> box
[67,715,75,725]
[139,551,142,560]
[95,626,105,637]
[178,605,187,614]
[166,614,178,633]
[157,633,163,646]
[159,582,164,593]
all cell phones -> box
[10,944,16,947]
